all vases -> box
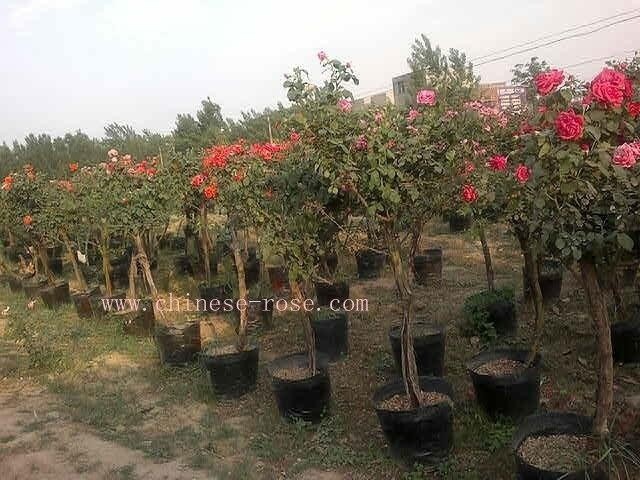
[512,411,610,480]
